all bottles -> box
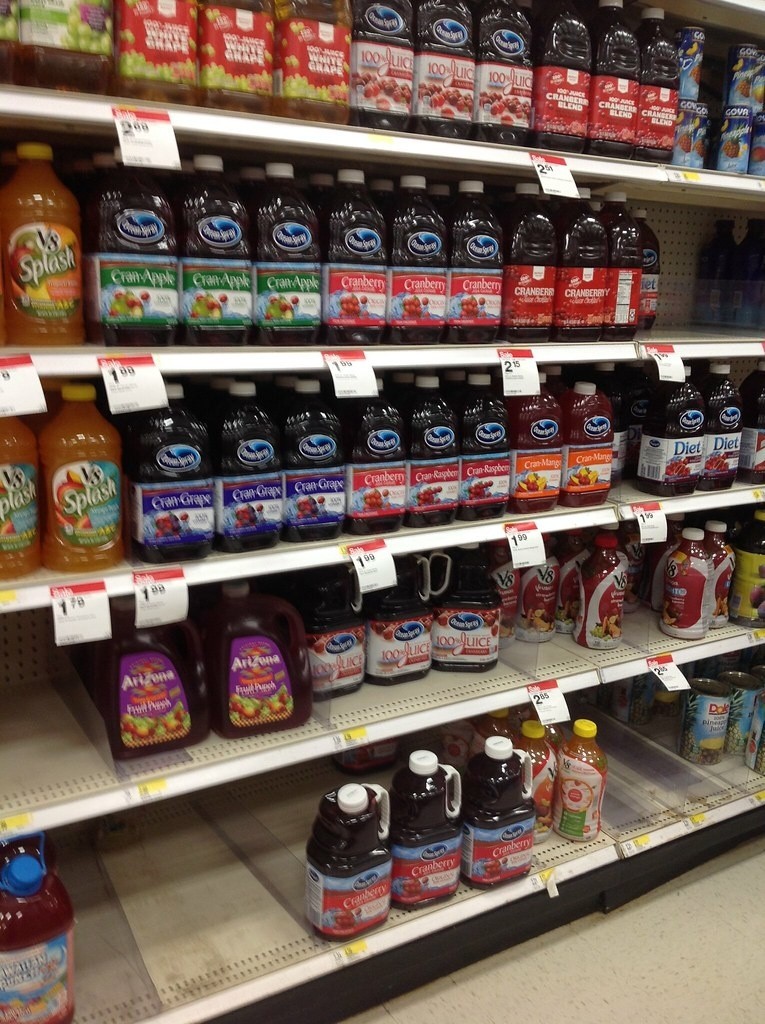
[0,829,74,1024]
[93,482,765,944]
[0,0,765,583]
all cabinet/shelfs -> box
[603,163,765,917]
[0,83,623,1024]
